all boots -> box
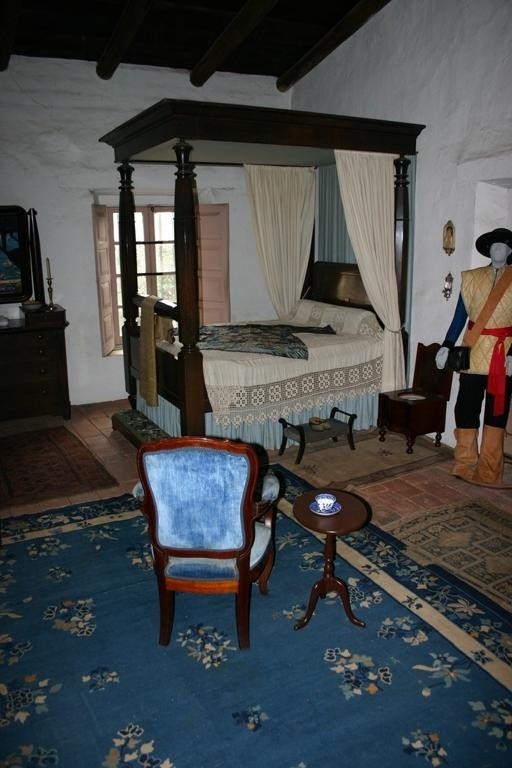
[472,423,505,485]
[449,428,479,479]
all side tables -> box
[294,488,372,630]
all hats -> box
[476,228,512,264]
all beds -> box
[100,99,426,456]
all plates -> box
[20,302,42,309]
[307,499,342,516]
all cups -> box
[315,493,336,511]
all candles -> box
[46,257,51,279]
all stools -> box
[278,407,356,464]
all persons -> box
[432,226,512,486]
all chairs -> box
[378,342,454,455]
[133,437,284,651]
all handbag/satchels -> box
[444,346,471,370]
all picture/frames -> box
[0,205,32,304]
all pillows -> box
[290,300,382,338]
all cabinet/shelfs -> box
[0,326,71,437]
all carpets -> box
[268,427,512,614]
[0,426,119,510]
[0,464,512,768]
